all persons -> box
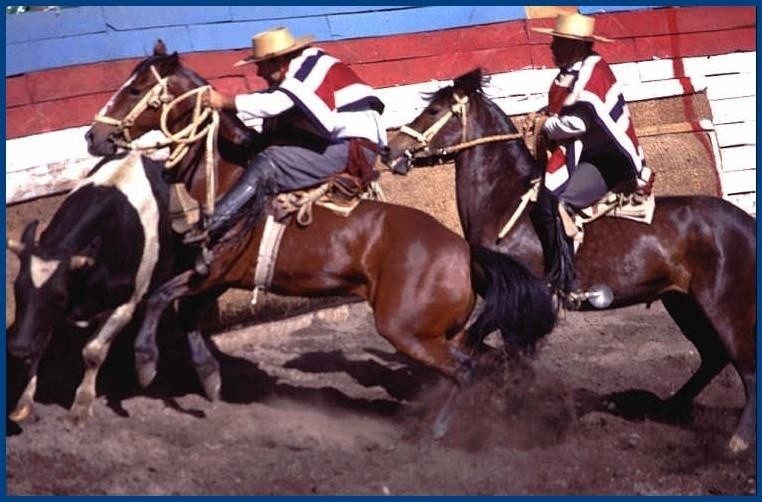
[171,27,387,249]
[521,12,655,293]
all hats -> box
[530,12,614,44]
[232,25,314,68]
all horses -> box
[6,149,224,423]
[380,65,756,457]
[84,38,558,442]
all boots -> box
[182,152,275,245]
